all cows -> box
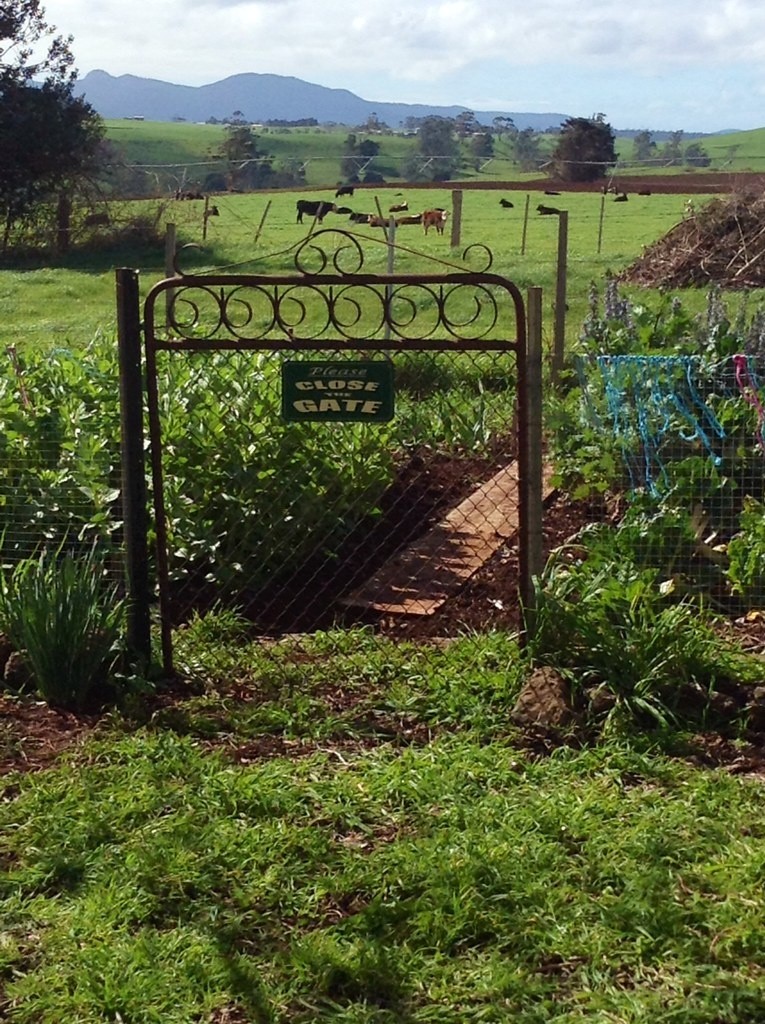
[174,188,220,217]
[296,186,451,237]
[498,189,651,217]
